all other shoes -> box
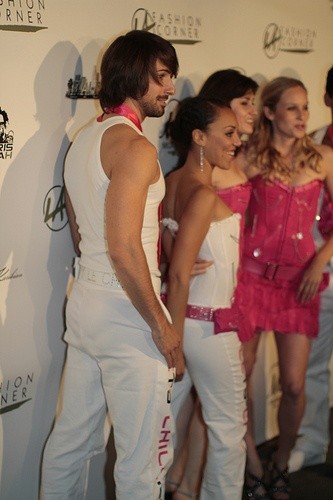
[243,469,270,500]
[269,461,293,500]
[164,477,181,500]
[171,487,199,500]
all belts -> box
[76,265,121,289]
[159,293,215,322]
[242,257,304,282]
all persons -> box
[39,30,182,500]
[158,69,259,500]
[233,77,333,500]
[289,67,333,473]
[159,96,249,500]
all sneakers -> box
[287,447,327,473]
